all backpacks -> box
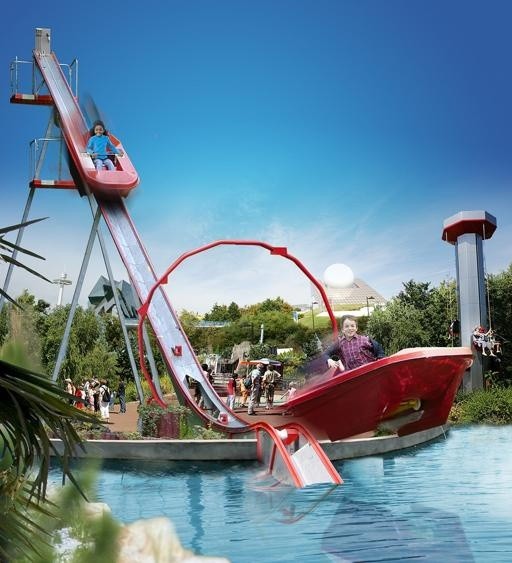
[101,387,110,402]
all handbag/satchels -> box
[243,376,252,390]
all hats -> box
[64,377,71,382]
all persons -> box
[201,363,210,408]
[118,378,128,415]
[195,368,214,404]
[226,372,238,412]
[474,325,497,357]
[260,363,281,410]
[238,374,249,408]
[323,312,384,373]
[245,363,264,416]
[63,376,115,424]
[87,121,123,171]
[280,380,297,401]
